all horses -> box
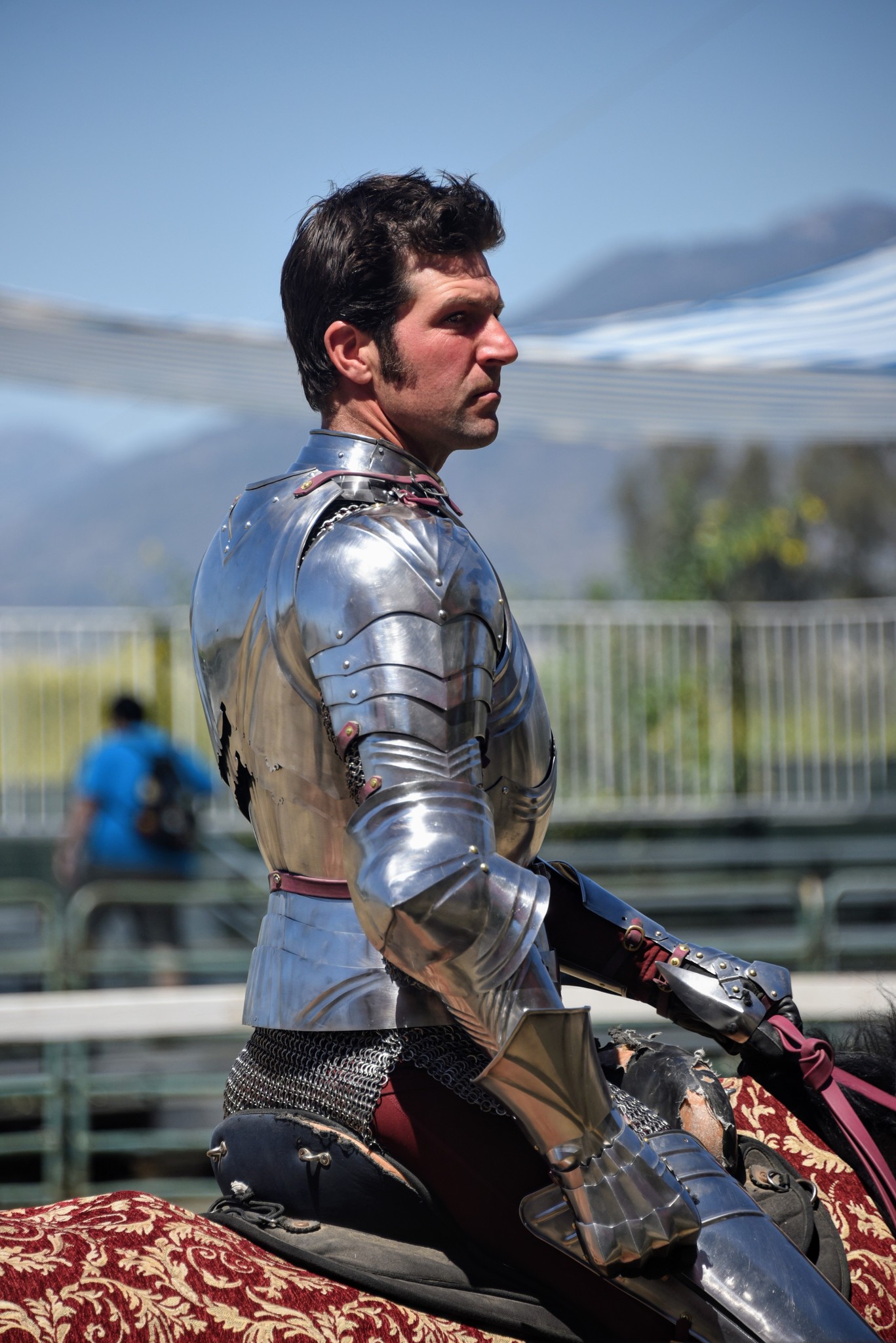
[0,982,896,1343]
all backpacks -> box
[107,741,197,849]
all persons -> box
[187,168,889,1343]
[50,691,216,1056]
